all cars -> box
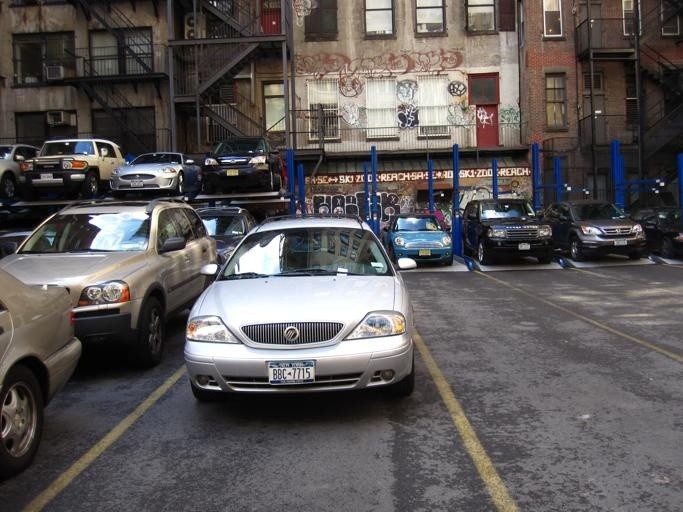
[2,139,284,201]
[183,214,414,403]
